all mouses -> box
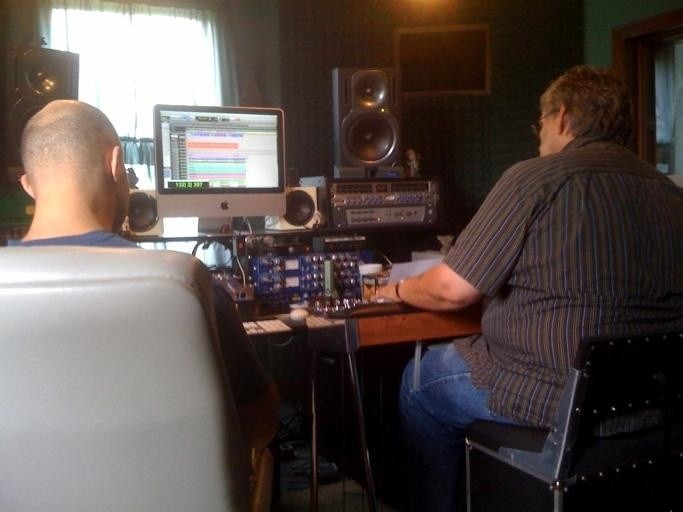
[293,306,309,322]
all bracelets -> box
[396,281,401,300]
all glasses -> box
[530,108,560,139]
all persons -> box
[7,98,281,476]
[370,63,683,512]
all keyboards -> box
[241,320,292,337]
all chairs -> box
[0,245,281,512]
[464,331,683,512]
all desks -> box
[241,304,484,512]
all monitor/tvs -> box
[153,106,288,238]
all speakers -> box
[265,185,317,231]
[332,63,403,180]
[128,189,165,239]
[1,46,79,182]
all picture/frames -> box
[392,22,493,99]
